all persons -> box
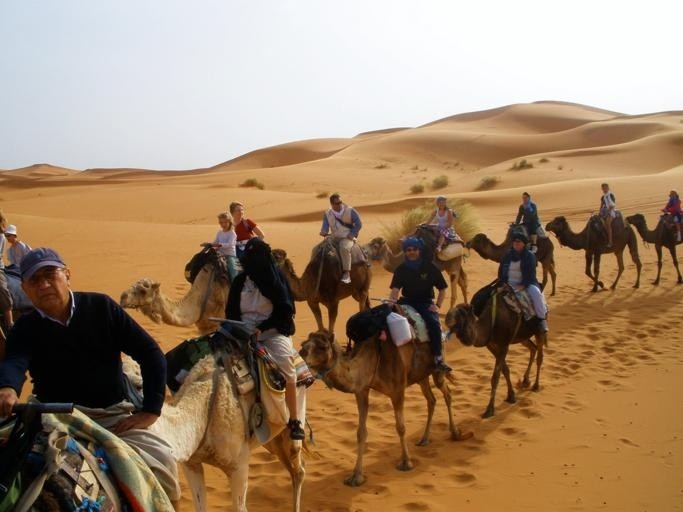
[319,196,362,284]
[386,236,451,370]
[0,216,15,326]
[426,196,453,252]
[660,190,682,242]
[599,184,616,248]
[231,202,263,244]
[5,223,29,265]
[0,249,183,511]
[219,237,305,440]
[200,213,237,282]
[515,193,540,253]
[501,234,548,334]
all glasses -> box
[5,234,11,237]
[28,269,64,286]
[334,201,342,205]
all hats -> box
[4,225,16,235]
[20,247,65,281]
[509,225,529,245]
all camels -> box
[626,213,683,286]
[118,262,229,337]
[298,303,460,487]
[445,285,548,419]
[271,243,371,331]
[363,223,468,308]
[544,211,642,293]
[466,223,556,296]
[121,331,314,512]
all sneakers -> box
[431,357,452,372]
[676,234,683,241]
[435,244,441,252]
[340,271,352,284]
[530,245,537,252]
[606,242,612,248]
[539,318,548,333]
[288,418,305,439]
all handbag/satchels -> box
[345,304,392,342]
[387,312,412,347]
[166,338,202,393]
[471,279,500,317]
[184,244,212,283]
[352,225,359,237]
